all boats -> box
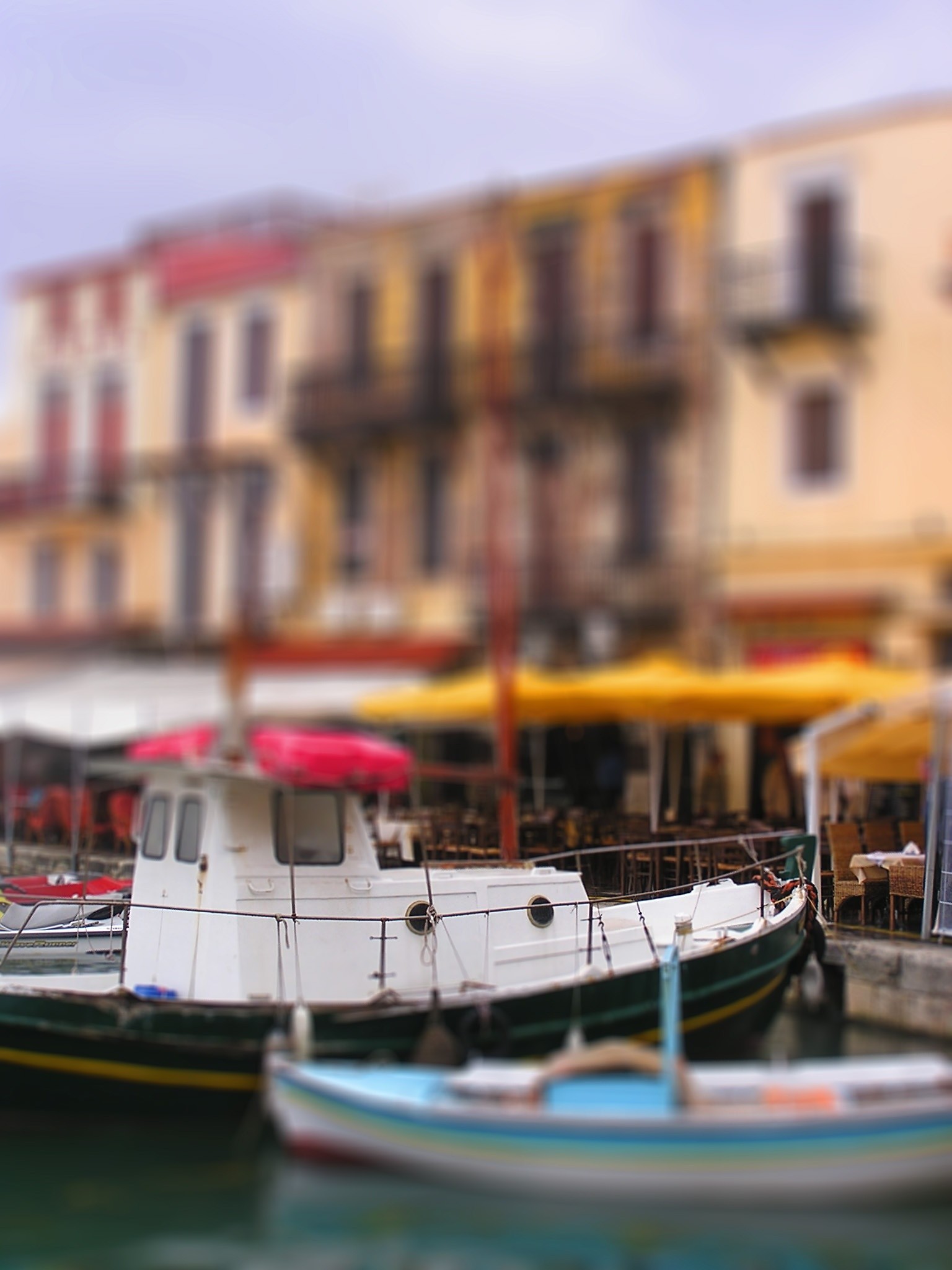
[0,724,820,1089]
[259,942,952,1206]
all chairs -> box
[0,770,137,858]
[364,801,925,938]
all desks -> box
[850,855,925,935]
[375,820,430,868]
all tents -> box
[793,682,952,938]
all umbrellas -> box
[356,651,930,829]
